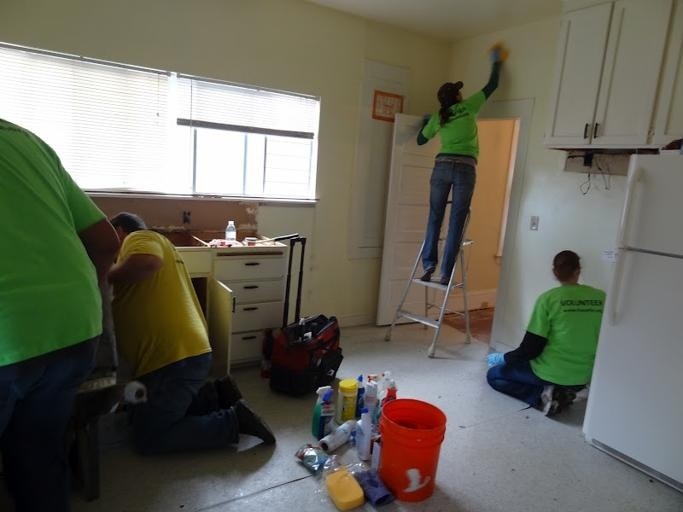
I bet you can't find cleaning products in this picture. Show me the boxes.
[356,373,365,415]
[382,371,399,408]
[356,406,373,463]
[365,374,381,416]
[319,419,358,453]
[311,384,337,441]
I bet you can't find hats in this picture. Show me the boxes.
[437,82,463,105]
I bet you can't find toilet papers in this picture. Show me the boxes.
[124,380,148,404]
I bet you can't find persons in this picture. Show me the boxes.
[413,42,504,288]
[0,116,121,512]
[104,210,277,457]
[481,248,606,419]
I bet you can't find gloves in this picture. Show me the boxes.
[487,353,506,368]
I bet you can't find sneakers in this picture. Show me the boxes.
[233,398,276,445]
[563,391,578,406]
[441,277,448,285]
[421,267,434,281]
[541,385,560,416]
[215,376,243,407]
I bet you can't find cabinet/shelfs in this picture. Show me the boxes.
[543,0,683,153]
[179,233,288,375]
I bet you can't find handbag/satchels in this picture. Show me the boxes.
[269,314,343,395]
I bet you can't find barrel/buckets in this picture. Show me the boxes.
[376,399,447,502]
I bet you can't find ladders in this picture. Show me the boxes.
[383,199,473,358]
